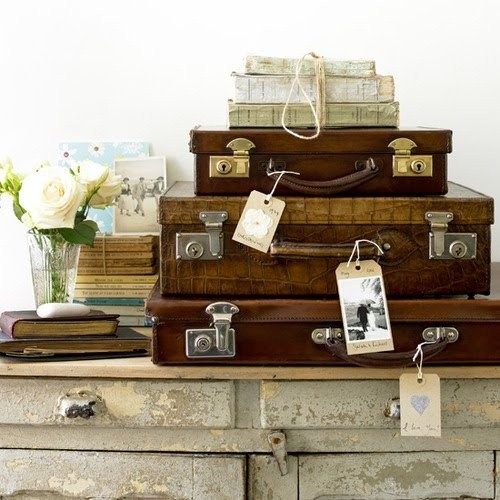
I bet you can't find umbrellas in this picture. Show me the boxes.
[357,299,376,306]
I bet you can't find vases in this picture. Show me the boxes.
[25,237,81,308]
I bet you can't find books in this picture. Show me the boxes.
[0,310,120,339]
[230,72,394,104]
[72,275,159,328]
[227,99,399,128]
[0,325,152,361]
[245,56,379,76]
[75,234,158,276]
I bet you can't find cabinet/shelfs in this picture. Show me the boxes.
[0,354,500,500]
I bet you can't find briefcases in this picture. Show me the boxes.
[146,276,499,368]
[157,181,494,297]
[187,125,453,195]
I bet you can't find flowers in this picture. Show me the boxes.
[0,154,116,303]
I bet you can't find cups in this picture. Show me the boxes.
[26,226,81,313]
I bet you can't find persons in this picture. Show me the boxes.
[151,177,165,220]
[366,305,376,330]
[357,304,370,332]
[132,177,148,216]
[118,177,135,216]
